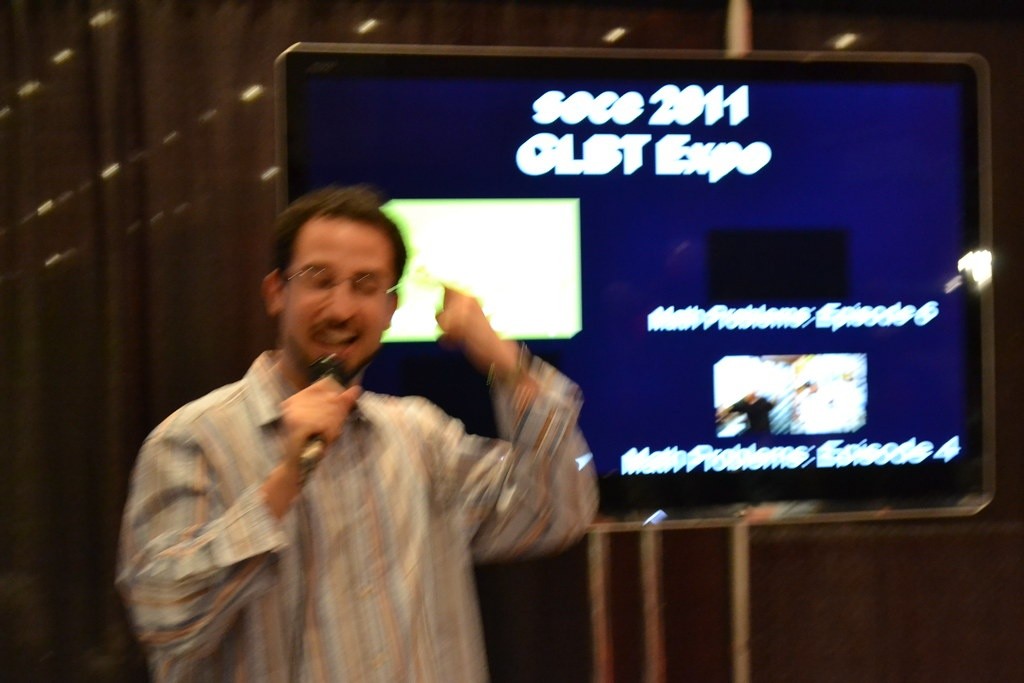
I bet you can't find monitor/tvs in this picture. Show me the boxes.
[280,54,994,533]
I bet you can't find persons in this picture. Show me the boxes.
[118,184,598,683]
[720,393,777,436]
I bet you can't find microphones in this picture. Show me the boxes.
[298,354,348,472]
